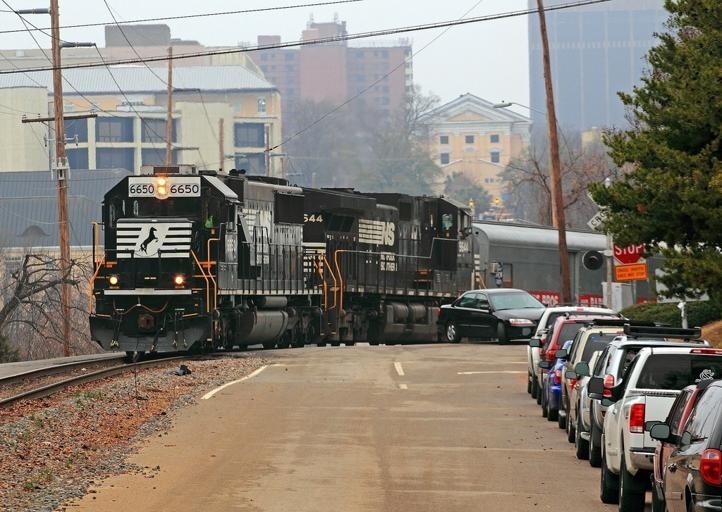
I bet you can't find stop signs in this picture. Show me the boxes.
[613,242,645,264]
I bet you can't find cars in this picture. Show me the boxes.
[437,288,546,344]
[527,307,722,512]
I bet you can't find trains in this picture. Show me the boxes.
[88,166,721,363]
